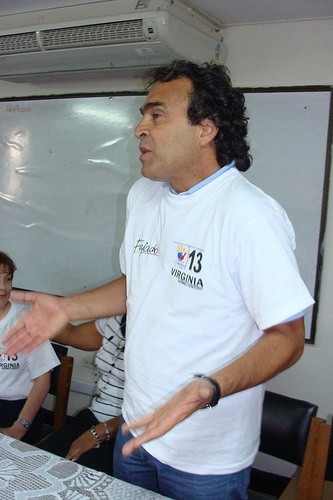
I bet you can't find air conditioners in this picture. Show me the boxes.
[0,0,227,81]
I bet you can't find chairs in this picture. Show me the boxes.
[23,342,75,448]
[248,389,333,500]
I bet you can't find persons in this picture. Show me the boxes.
[2,59,316,500]
[0,253,62,443]
[49,313,126,476]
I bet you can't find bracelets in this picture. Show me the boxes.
[90,427,101,448]
[102,421,110,440]
[17,418,30,429]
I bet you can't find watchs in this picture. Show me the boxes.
[194,374,220,409]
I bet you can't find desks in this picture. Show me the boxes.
[0,432,173,499]
